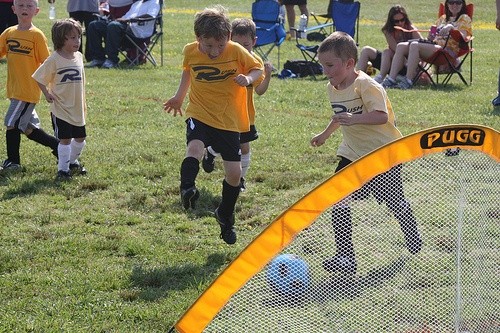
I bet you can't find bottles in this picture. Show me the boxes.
[49,2,55,19]
[299,13,307,38]
[431,25,436,38]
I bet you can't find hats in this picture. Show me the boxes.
[281,69,296,78]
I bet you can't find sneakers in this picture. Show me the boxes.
[239,177,246,192]
[323,260,357,275]
[57,170,72,181]
[202,148,216,173]
[180,187,199,211]
[51,152,59,163]
[214,209,237,245]
[0,161,21,170]
[405,233,422,254]
[382,78,397,88]
[394,80,413,88]
[69,164,86,174]
[374,77,383,83]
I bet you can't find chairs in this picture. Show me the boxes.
[92,0,163,67]
[252,0,287,75]
[291,0,360,80]
[409,3,473,89]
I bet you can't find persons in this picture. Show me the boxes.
[308,31,421,275]
[382,0,471,89]
[0,0,59,170]
[163,8,262,245]
[278,0,308,38]
[355,6,423,84]
[30,17,87,179]
[492,0,500,109]
[202,18,273,192]
[66,0,161,69]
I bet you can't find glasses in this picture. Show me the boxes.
[394,19,406,23]
[448,1,463,5]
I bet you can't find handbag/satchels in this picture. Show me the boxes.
[284,60,323,76]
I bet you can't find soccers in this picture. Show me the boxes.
[267,254,310,298]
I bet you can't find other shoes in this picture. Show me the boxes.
[493,96,500,105]
[101,60,119,69]
[84,60,104,68]
